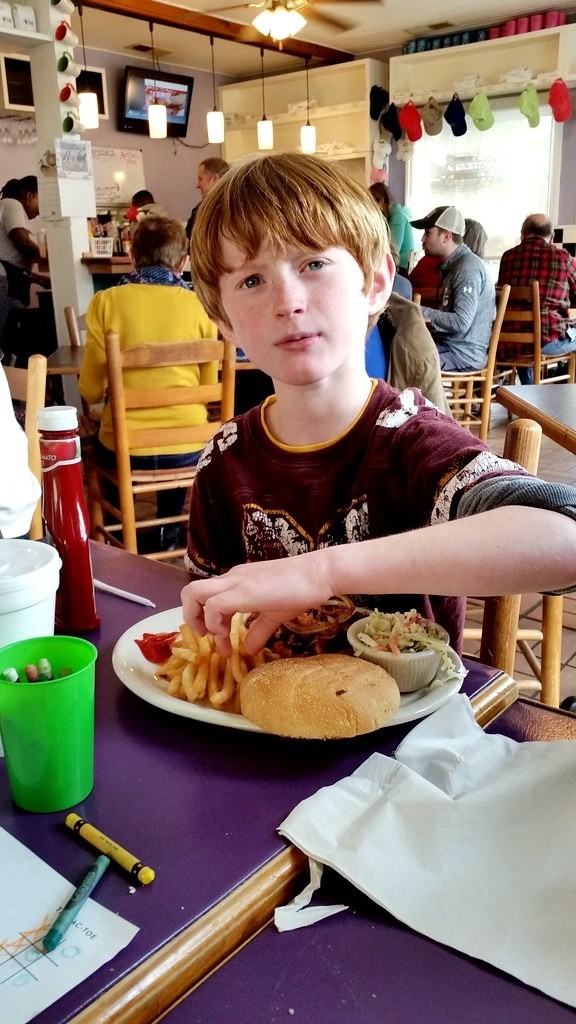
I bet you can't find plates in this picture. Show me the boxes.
[112,606,465,733]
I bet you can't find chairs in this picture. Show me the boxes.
[1,354,48,540]
[493,279,576,420]
[376,291,453,418]
[438,284,512,443]
[81,328,236,561]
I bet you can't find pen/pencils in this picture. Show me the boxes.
[92,578,155,608]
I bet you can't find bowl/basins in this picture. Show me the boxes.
[348,615,450,693]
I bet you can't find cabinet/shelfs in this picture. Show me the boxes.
[217,58,389,188]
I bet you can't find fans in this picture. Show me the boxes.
[178,0,384,43]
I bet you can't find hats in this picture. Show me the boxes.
[370,167,388,184]
[372,138,392,171]
[410,205,466,237]
[469,90,494,131]
[370,85,390,121]
[381,103,402,143]
[397,136,415,161]
[419,95,443,135]
[443,92,467,136]
[518,83,540,128]
[548,77,572,122]
[399,100,423,141]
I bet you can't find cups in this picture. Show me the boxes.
[0,539,63,647]
[1,635,99,813]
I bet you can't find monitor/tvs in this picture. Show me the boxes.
[119,66,195,138]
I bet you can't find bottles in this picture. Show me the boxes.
[121,222,131,257]
[37,405,101,629]
[38,227,48,258]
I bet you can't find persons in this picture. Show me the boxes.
[0,363,41,539]
[145,88,184,116]
[497,213,576,384]
[77,217,218,551]
[408,219,488,330]
[0,175,46,367]
[179,151,576,661]
[129,190,167,238]
[186,157,229,259]
[409,206,494,373]
[369,182,413,277]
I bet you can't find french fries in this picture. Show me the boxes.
[154,611,268,714]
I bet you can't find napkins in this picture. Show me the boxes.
[276,692,576,1008]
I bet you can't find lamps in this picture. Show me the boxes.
[147,21,167,140]
[77,4,100,130]
[252,0,311,42]
[256,48,273,150]
[205,36,224,143]
[299,59,316,153]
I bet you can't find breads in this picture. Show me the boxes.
[238,653,399,739]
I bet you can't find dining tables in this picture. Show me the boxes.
[148,694,576,1024]
[0,537,518,1024]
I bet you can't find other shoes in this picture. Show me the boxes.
[153,521,185,561]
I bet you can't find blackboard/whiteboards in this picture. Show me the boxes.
[92,145,147,207]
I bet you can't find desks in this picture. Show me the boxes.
[496,384,576,456]
[46,344,259,376]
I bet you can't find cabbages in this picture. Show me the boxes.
[358,610,469,692]
